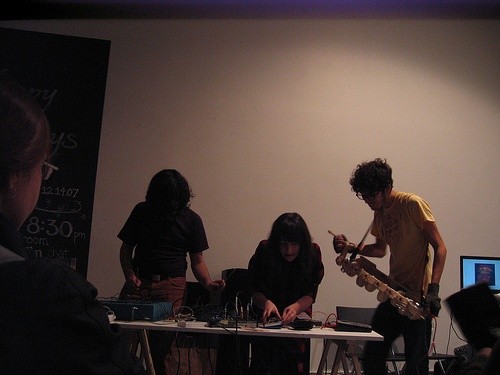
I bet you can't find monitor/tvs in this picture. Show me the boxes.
[460,256,500,294]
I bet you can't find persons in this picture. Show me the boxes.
[0,77,141,375]
[247,212,325,375]
[333,159,447,375]
[117,168,226,375]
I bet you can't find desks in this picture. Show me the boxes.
[109,315,384,375]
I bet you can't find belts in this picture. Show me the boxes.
[134,269,185,281]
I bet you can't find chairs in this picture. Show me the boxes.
[177,282,228,349]
[221,267,261,319]
[336,306,409,374]
[422,341,462,375]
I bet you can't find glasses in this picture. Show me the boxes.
[356,190,381,201]
[36,157,59,179]
